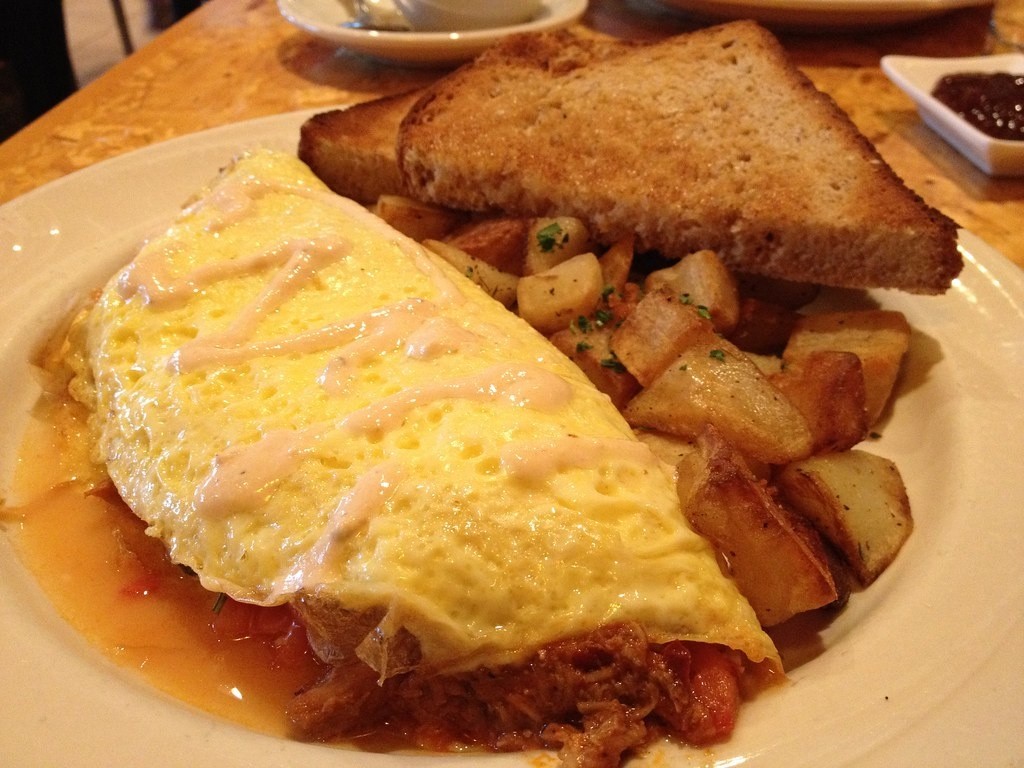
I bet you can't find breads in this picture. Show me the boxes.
[297,86,425,203]
[398,20,963,296]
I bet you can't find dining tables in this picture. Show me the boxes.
[0,0,1024,269]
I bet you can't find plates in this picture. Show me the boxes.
[278,0,590,62]
[2,101,1024,768]
[880,53,1024,175]
